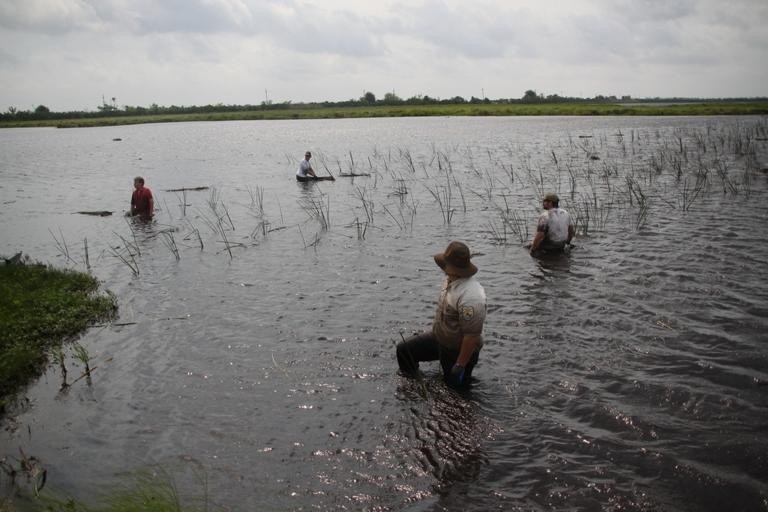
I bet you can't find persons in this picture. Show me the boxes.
[295,151,317,182]
[522,191,576,254]
[396,241,486,385]
[402,395,486,508]
[130,176,153,218]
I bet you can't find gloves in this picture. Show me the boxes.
[313,175,318,180]
[529,245,539,258]
[446,363,466,387]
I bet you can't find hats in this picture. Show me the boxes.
[537,192,559,202]
[304,151,311,157]
[434,241,478,278]
